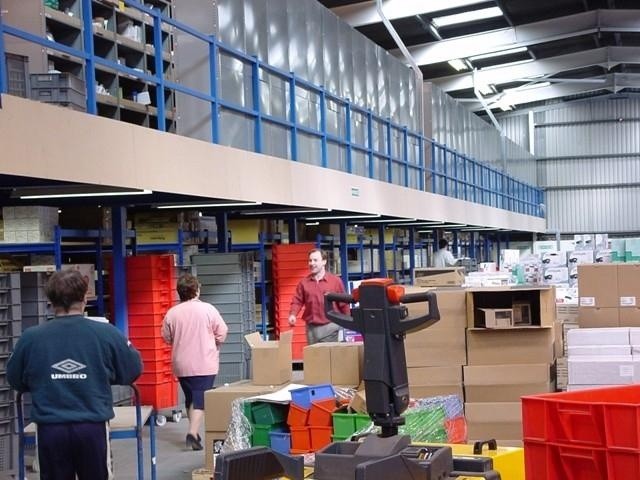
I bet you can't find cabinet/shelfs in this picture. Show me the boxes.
[41,0,176,134]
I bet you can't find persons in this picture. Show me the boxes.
[6,269,144,480]
[432,238,460,267]
[287,248,351,345]
[161,273,228,451]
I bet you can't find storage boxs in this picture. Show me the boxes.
[30,73,87,113]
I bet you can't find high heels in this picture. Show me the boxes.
[186,433,202,451]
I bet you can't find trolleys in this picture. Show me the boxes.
[13,382,163,480]
[132,400,182,426]
